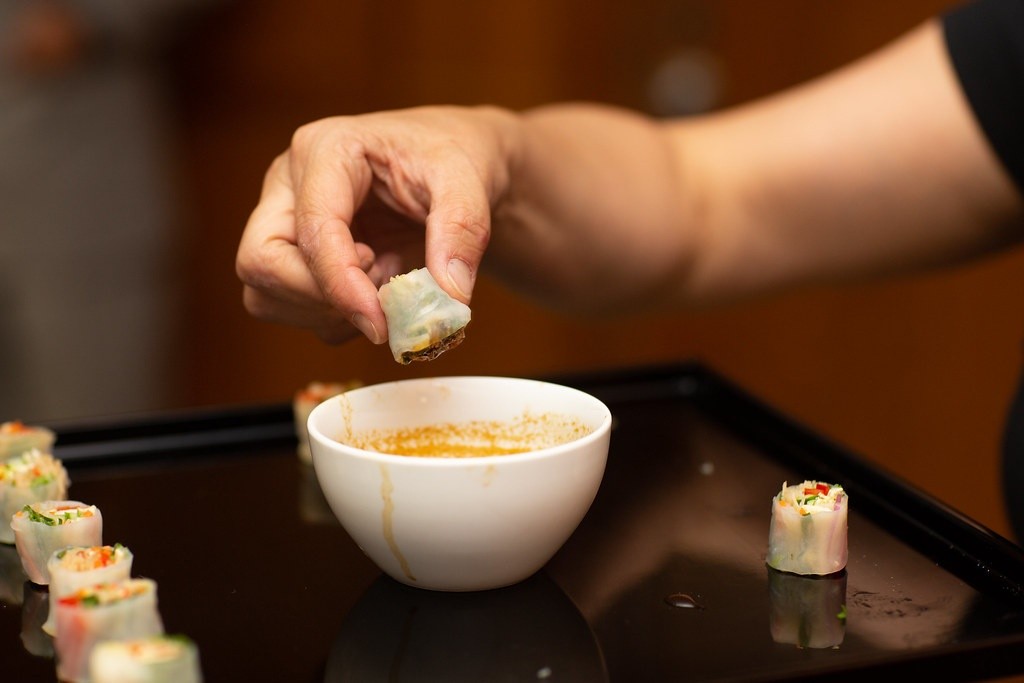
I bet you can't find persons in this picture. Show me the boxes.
[235,0,1024,553]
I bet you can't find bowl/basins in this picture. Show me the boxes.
[306,377,611,591]
